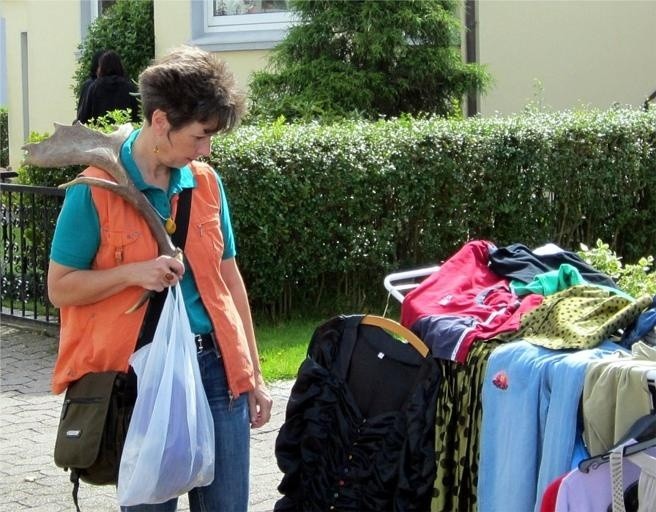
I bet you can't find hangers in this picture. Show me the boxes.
[361,289,428,358]
[577,414,656,475]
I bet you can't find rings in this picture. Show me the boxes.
[163,270,175,284]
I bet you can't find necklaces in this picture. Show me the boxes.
[138,189,177,237]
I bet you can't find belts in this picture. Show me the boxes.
[193,334,214,355]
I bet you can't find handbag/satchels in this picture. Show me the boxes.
[54,364,137,486]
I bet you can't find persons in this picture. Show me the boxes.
[45,45,274,512]
[71,49,140,125]
[75,49,103,117]
